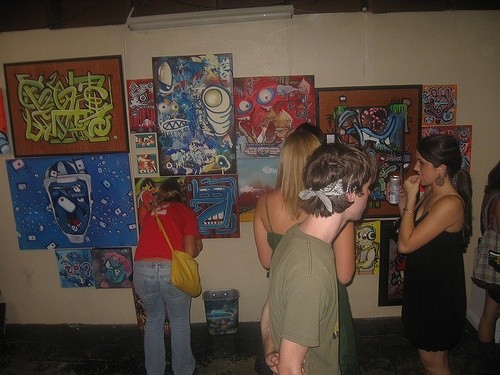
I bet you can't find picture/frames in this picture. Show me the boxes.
[315,84,422,218]
[3,54,130,158]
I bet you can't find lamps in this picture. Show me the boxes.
[127,4,294,31]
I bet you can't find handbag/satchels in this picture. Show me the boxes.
[172,250,202,298]
[471,195,500,291]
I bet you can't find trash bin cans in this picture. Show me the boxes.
[203,289,241,335]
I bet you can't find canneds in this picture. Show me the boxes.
[389,175,400,204]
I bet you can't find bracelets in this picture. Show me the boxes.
[404,208,414,216]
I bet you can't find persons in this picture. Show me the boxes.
[385,134,472,375]
[133,177,203,375]
[253,129,359,375]
[472,161,500,375]
[259,140,379,375]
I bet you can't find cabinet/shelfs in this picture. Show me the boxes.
[378,220,408,307]
